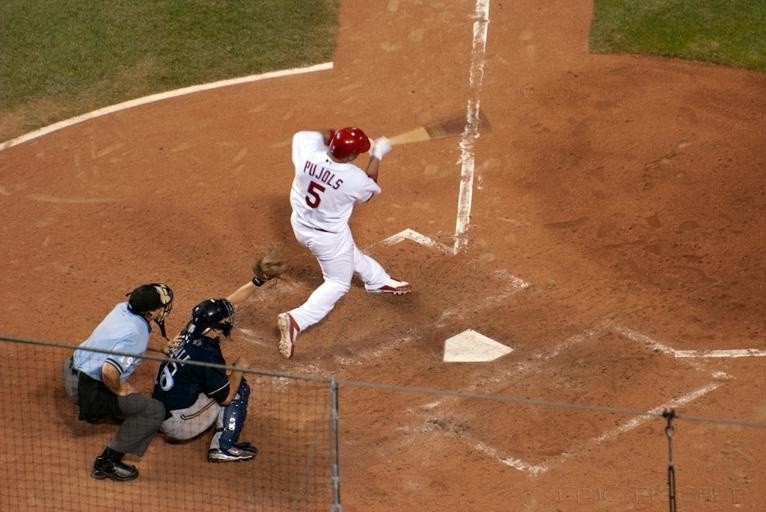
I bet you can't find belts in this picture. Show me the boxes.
[69,355,77,376]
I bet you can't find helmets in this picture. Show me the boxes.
[192,297,235,335]
[329,127,371,160]
[124,282,174,312]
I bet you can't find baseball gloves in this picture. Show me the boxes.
[253,254,288,282]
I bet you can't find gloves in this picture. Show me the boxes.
[367,136,392,161]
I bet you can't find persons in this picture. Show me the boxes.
[157,254,289,461]
[276,127,411,359]
[63,284,184,481]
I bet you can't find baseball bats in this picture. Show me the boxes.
[388,108,491,154]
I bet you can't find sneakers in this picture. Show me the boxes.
[208,447,253,463]
[277,312,299,359]
[368,277,412,296]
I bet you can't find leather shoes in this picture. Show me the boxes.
[92,456,139,481]
